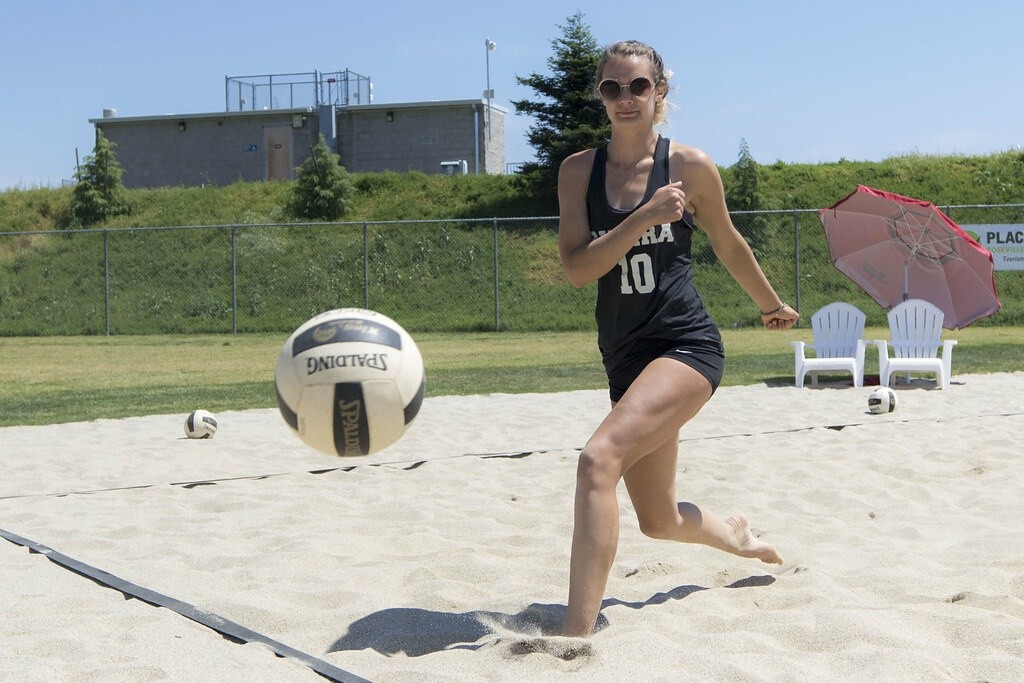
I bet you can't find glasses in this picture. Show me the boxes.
[596,76,657,101]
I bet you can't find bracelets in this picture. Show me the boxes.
[761,302,786,316]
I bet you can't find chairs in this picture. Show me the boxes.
[873,299,958,390]
[790,301,873,388]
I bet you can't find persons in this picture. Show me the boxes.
[558,39,800,638]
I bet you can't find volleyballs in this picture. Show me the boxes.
[274,306,426,458]
[868,387,900,414]
[184,409,217,439]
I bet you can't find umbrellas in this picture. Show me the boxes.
[817,183,1003,383]
